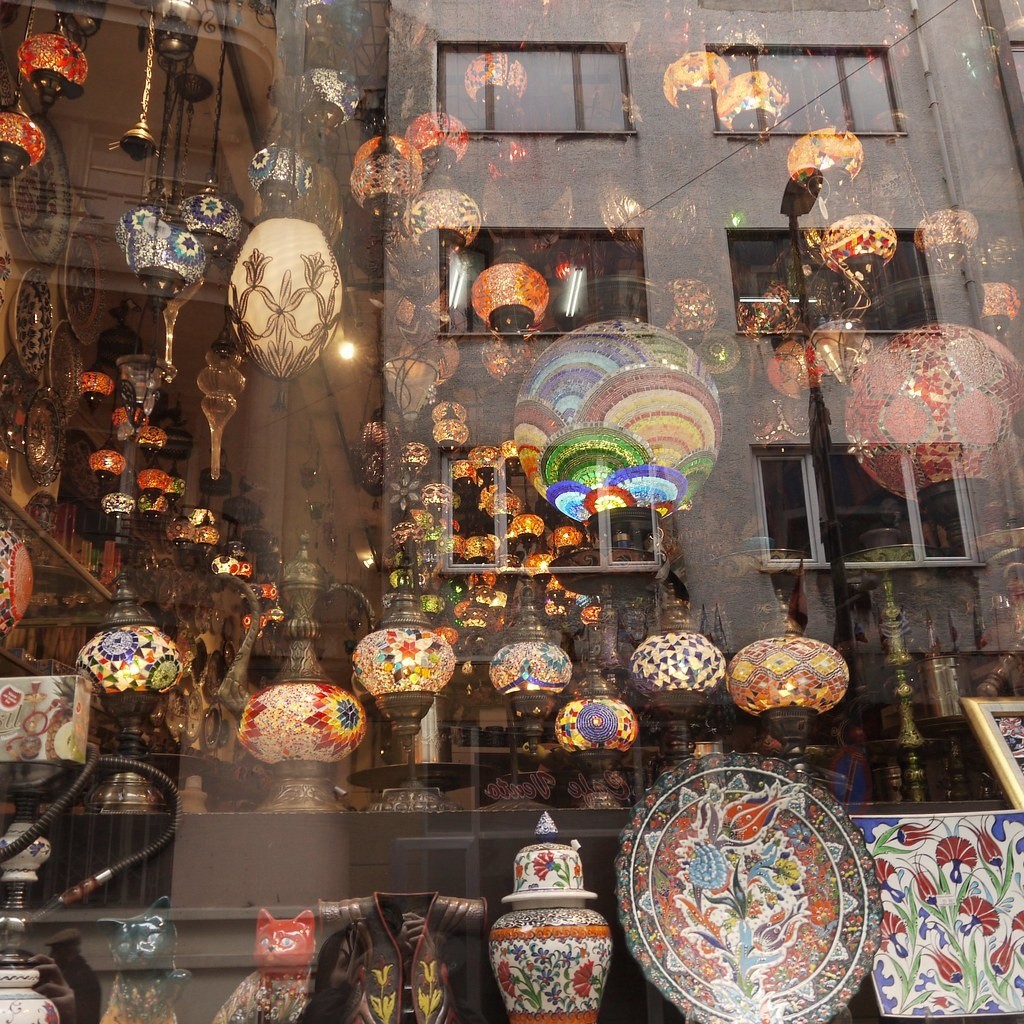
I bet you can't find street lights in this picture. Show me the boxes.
[778,165,866,721]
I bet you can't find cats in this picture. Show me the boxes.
[212,909,316,1024]
[97,896,190,1024]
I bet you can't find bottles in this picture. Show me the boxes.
[488,811,613,1024]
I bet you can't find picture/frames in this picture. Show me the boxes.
[959,694,1024,811]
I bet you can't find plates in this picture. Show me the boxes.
[614,750,880,1024]
[849,809,1024,1017]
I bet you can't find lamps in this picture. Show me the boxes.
[0,0,1024,811]
[740,296,818,304]
[449,261,467,310]
[565,268,584,318]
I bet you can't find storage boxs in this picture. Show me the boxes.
[0,674,93,766]
[8,647,40,670]
[38,658,77,676]
